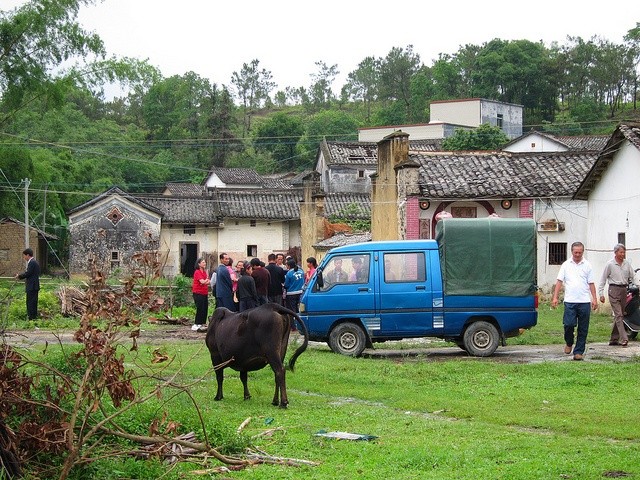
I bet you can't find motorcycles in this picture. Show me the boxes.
[610,267,640,341]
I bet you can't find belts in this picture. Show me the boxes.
[610,284,628,287]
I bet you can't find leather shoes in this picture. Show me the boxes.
[574,354,583,360]
[565,346,572,353]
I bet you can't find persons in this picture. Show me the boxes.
[259,261,265,267]
[216,253,234,312]
[276,253,287,270]
[600,243,640,347]
[15,249,40,319]
[264,254,285,304]
[384,260,395,281]
[192,258,210,330]
[250,258,268,304]
[235,260,245,277]
[283,257,304,330]
[324,256,348,286]
[210,268,217,297]
[236,262,258,312]
[281,258,294,307]
[226,257,239,302]
[350,257,362,281]
[304,257,318,285]
[551,242,598,360]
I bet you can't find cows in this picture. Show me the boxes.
[205,303,309,409]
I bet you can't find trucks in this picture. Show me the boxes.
[294,218,539,358]
